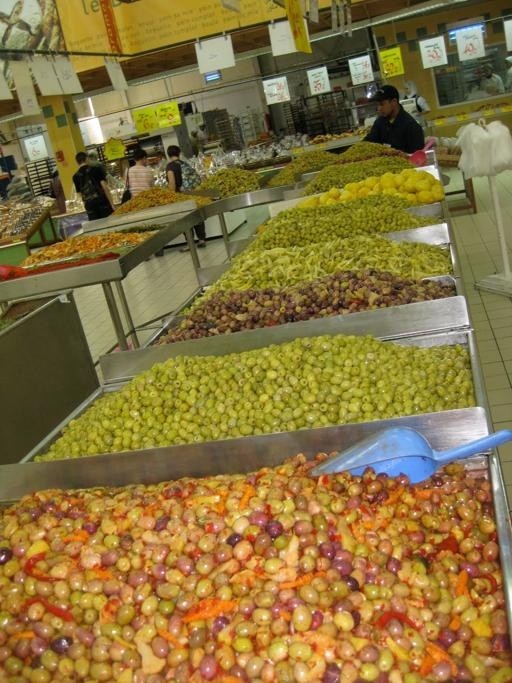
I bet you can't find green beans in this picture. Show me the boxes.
[179,192,453,316]
[34,335,476,462]
[0,452,512,683]
[110,186,213,206]
[278,141,416,195]
[195,168,262,198]
[149,267,456,346]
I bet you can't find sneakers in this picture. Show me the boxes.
[179,242,207,252]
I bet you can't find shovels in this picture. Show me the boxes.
[304,427,512,486]
[410,139,436,166]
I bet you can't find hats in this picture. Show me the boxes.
[367,85,398,102]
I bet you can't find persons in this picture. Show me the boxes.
[191,123,209,157]
[73,150,116,221]
[480,56,512,96]
[361,79,430,154]
[51,170,66,214]
[166,146,206,252]
[121,150,163,261]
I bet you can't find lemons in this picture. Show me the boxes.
[296,168,446,208]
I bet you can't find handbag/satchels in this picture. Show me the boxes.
[119,189,132,206]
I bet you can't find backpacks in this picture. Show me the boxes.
[174,160,201,196]
[76,167,101,202]
